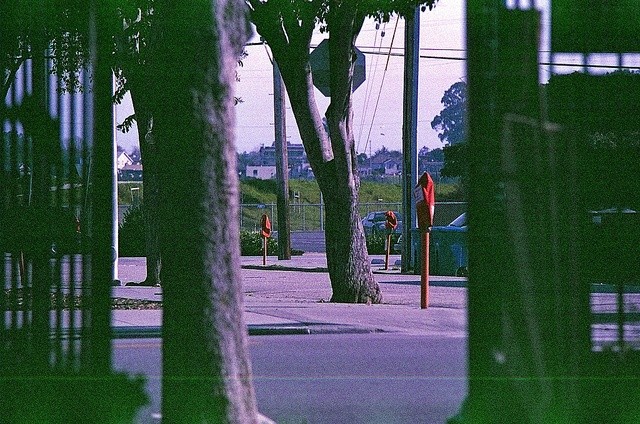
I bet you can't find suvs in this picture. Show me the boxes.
[0,206,81,258]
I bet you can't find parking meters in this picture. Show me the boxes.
[385,210,398,270]
[413,173,434,308]
[260,214,272,263]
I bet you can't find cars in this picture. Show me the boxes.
[395,211,468,264]
[362,211,403,240]
